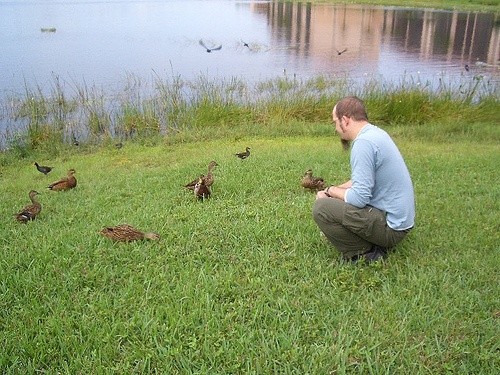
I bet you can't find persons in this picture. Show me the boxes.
[313,94,416,260]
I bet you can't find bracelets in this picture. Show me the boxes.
[325,185,335,199]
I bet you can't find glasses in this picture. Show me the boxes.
[330,116,350,126]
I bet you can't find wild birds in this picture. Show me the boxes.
[199,41,222,53]
[12,190,42,224]
[300,170,324,191]
[194,174,212,200]
[33,161,55,175]
[241,41,250,48]
[97,224,161,242]
[46,169,80,192]
[182,161,219,190]
[235,147,251,159]
[333,47,347,56]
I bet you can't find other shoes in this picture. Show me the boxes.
[352,246,386,264]
